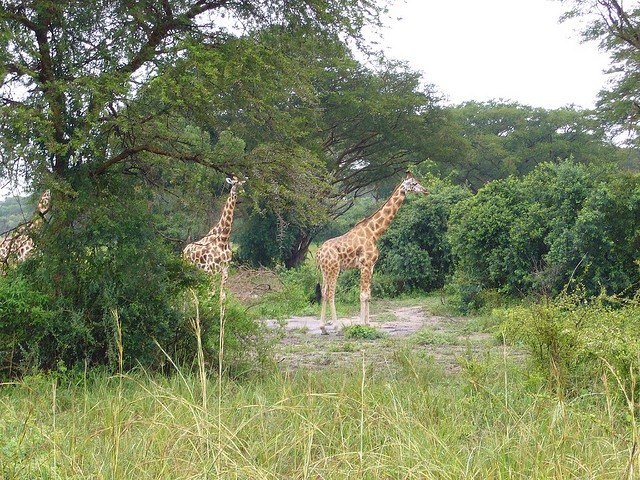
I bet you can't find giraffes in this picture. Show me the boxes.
[315,169,430,336]
[0,183,61,278]
[181,172,248,302]
